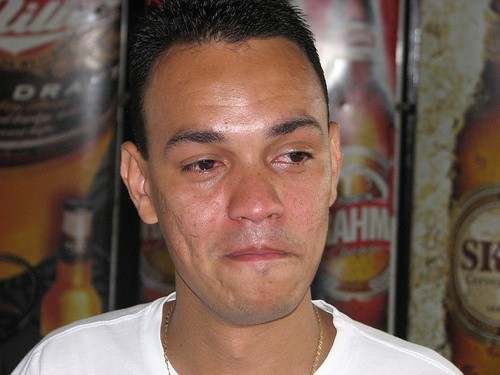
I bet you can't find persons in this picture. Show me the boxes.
[9,1,466,375]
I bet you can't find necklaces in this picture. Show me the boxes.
[163,301,323,375]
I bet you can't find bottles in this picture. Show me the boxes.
[440,0,500,375]
[38,198,101,344]
[304,1,397,341]
